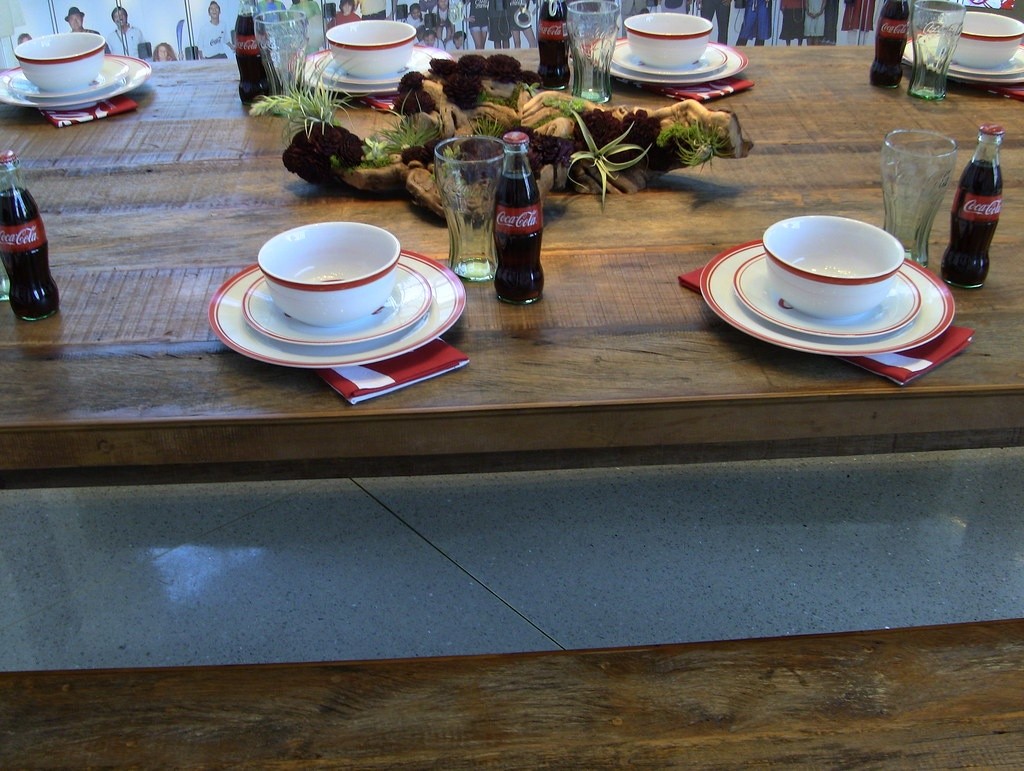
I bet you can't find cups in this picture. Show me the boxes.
[434,134,505,282]
[566,0,621,105]
[0,159,26,300]
[880,129,958,268]
[907,0,966,101]
[253,10,306,98]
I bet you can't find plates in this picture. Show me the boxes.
[0,54,152,111]
[898,40,1024,85]
[207,250,466,369]
[588,37,748,86]
[699,241,956,357]
[301,44,454,95]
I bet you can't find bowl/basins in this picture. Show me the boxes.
[256,222,402,329]
[624,12,714,68]
[325,20,417,78]
[13,32,105,92]
[761,215,905,322]
[938,12,1024,70]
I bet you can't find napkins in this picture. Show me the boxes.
[902,39,1023,103]
[613,78,769,105]
[675,266,982,388]
[316,334,472,405]
[38,95,138,130]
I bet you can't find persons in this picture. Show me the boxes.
[198,1,235,59]
[152,42,177,62]
[18,33,32,45]
[621,0,732,44]
[405,0,466,50]
[462,0,539,49]
[253,0,398,52]
[841,0,876,46]
[65,6,111,54]
[736,0,826,46]
[107,7,143,58]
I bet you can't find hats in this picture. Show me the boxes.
[65,7,85,21]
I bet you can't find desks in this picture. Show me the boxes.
[1,38,1024,495]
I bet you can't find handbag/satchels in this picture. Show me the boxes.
[734,0,746,8]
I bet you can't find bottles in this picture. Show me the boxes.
[870,0,910,89]
[493,131,545,304]
[234,0,281,107]
[940,122,1006,289]
[536,0,571,91]
[0,151,59,320]
